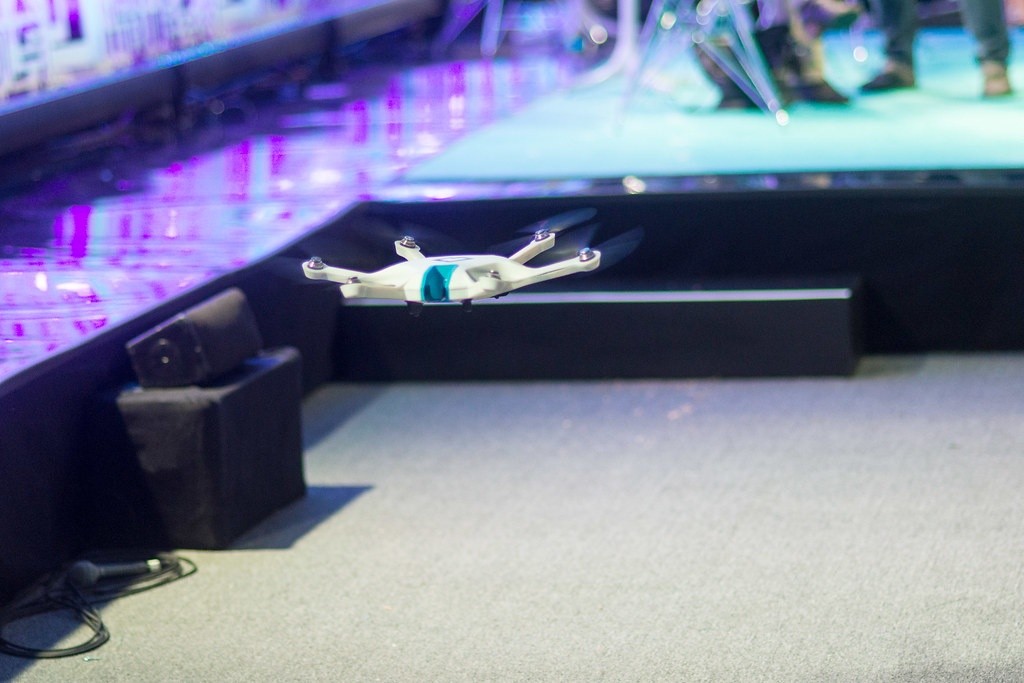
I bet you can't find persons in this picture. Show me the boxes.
[694,0,847,112]
[856,0,1011,100]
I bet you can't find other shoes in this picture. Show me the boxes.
[795,81,848,103]
[719,93,755,108]
[983,60,1013,97]
[860,61,914,92]
